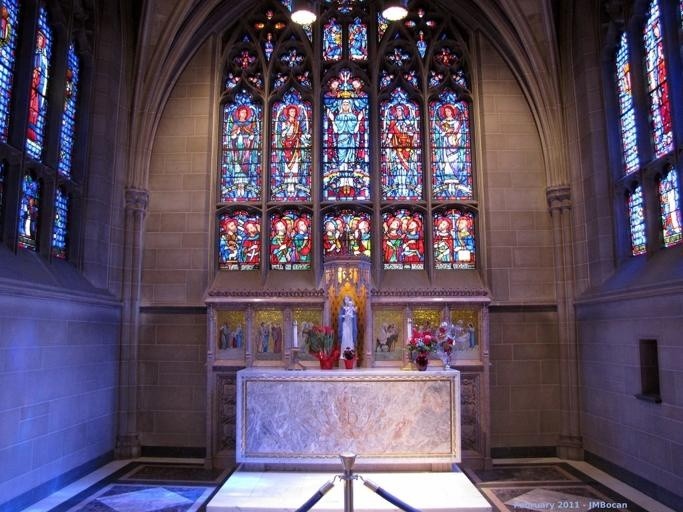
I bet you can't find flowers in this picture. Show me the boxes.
[407,322,458,357]
[303,323,355,359]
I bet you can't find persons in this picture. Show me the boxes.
[278,104,308,194]
[339,296,359,359]
[434,107,467,194]
[326,97,366,174]
[217,316,477,352]
[227,106,255,177]
[221,211,475,266]
[383,103,419,199]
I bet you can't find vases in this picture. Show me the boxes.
[318,356,354,369]
[412,351,428,371]
